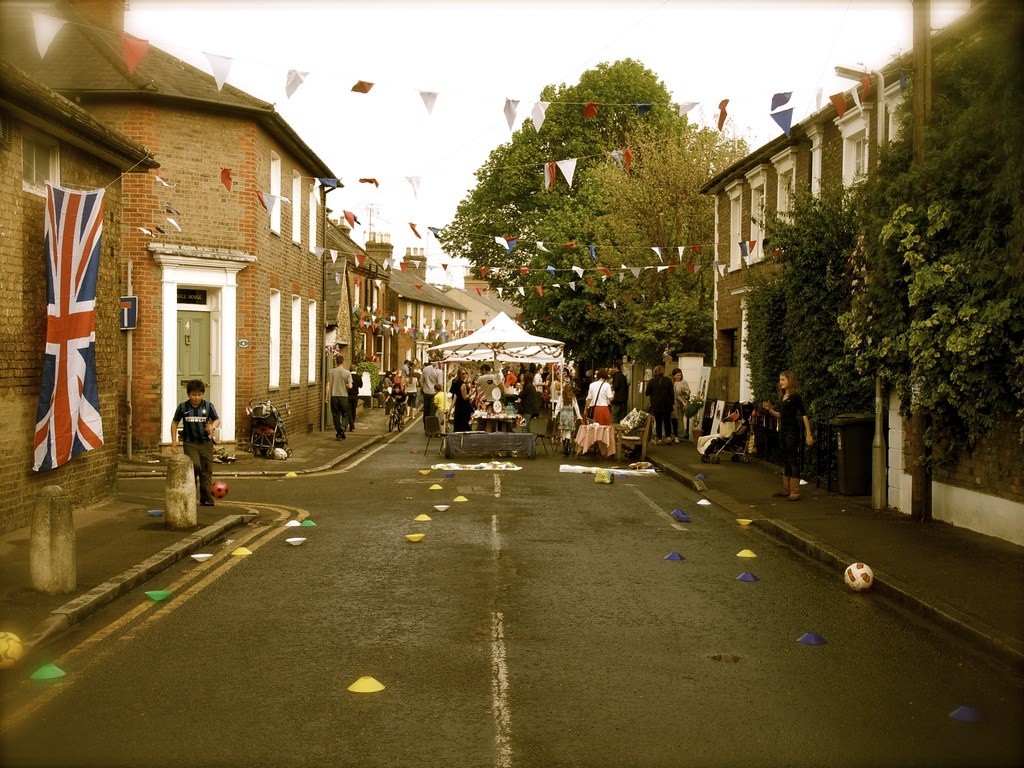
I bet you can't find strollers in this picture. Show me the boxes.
[696,400,757,464]
[247,396,292,459]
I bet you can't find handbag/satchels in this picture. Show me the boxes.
[586,406,595,419]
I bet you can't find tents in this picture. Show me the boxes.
[435,346,564,420]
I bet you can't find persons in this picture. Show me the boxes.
[325,355,363,441]
[504,373,520,433]
[171,380,221,508]
[473,364,504,433]
[645,365,675,446]
[517,363,629,428]
[449,367,476,433]
[762,370,813,501]
[419,363,444,437]
[611,362,628,423]
[670,368,691,444]
[552,383,583,456]
[373,357,443,428]
[434,384,451,437]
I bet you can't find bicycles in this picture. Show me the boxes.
[385,401,405,434]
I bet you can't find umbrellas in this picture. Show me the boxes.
[426,310,565,373]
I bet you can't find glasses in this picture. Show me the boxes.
[462,372,468,375]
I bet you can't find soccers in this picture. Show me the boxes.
[844,562,874,592]
[211,481,228,498]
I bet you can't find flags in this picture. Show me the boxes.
[31,178,107,473]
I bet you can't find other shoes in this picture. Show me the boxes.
[665,438,672,444]
[653,440,662,445]
[350,428,355,432]
[674,436,680,444]
[335,434,341,438]
[201,500,213,506]
[339,428,346,440]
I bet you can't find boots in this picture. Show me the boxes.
[773,474,791,497]
[787,477,801,501]
[561,438,570,455]
